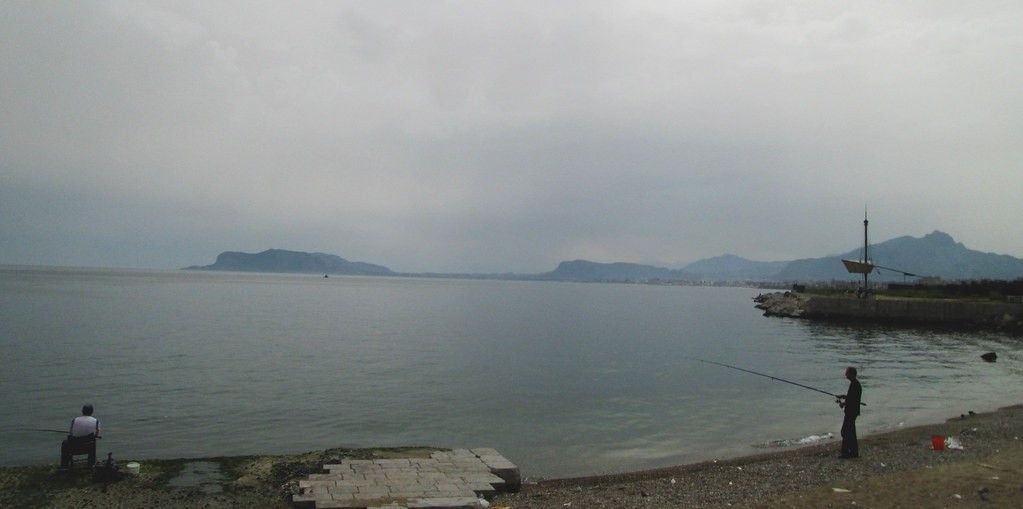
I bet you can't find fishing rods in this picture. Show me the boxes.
[686,354,866,407]
[1,429,102,438]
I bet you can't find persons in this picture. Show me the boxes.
[836,366,862,460]
[857,287,867,298]
[59,403,102,470]
[865,288,875,299]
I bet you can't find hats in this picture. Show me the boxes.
[83,403,93,411]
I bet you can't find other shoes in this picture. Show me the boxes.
[89,461,97,468]
[849,454,859,458]
[57,465,69,470]
[842,455,849,458]
